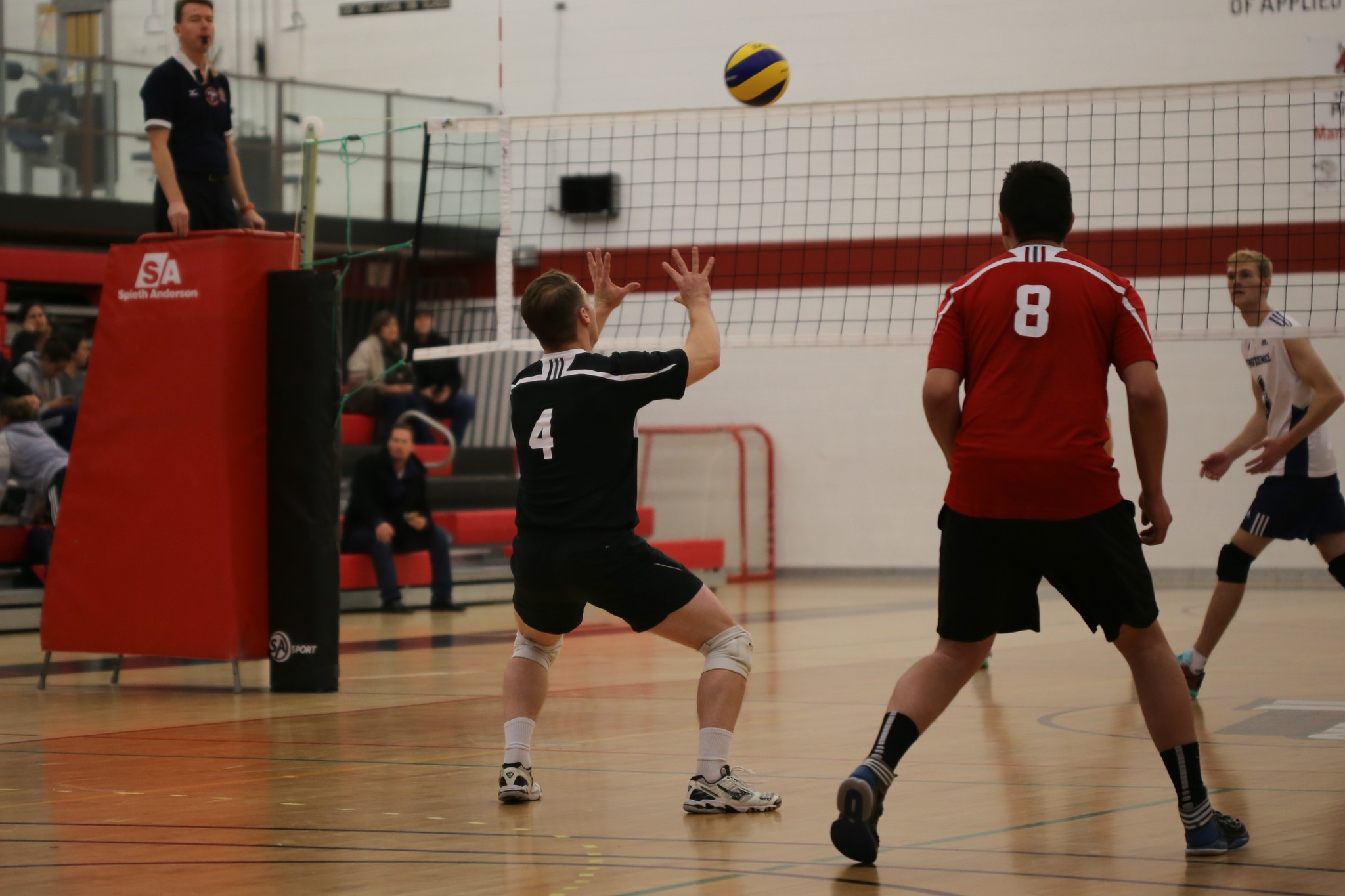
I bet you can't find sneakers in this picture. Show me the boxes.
[498,761,542,803]
[1184,807,1249,856]
[683,765,781,814]
[1173,649,1206,697]
[831,764,886,863]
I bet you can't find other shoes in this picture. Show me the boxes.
[430,597,465,612]
[380,600,414,614]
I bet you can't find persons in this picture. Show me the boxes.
[1173,250,1345,698]
[1,0,477,614]
[830,159,1251,865]
[499,246,784,816]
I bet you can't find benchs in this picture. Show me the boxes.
[0,345,724,591]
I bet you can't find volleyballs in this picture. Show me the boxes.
[724,41,790,106]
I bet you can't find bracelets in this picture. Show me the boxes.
[240,202,254,213]
